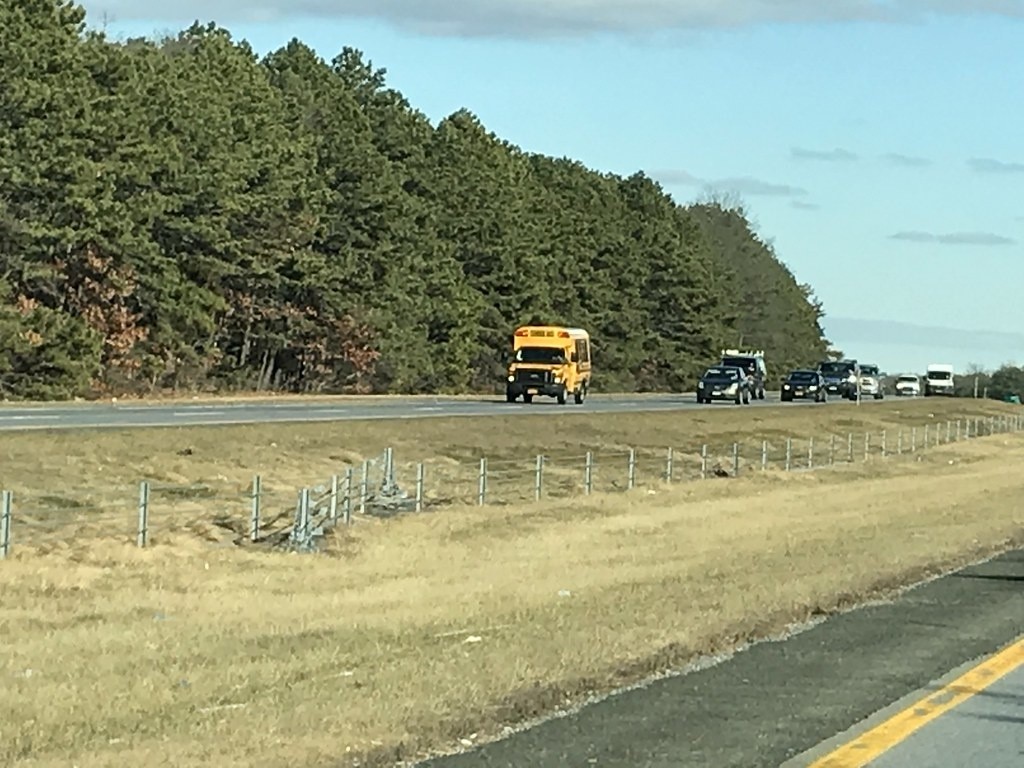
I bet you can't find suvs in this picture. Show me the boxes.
[719,348,767,400]
[816,360,862,402]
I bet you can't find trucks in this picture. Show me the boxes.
[923,365,956,396]
[504,325,591,405]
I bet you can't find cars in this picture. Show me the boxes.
[780,370,829,403]
[695,366,754,405]
[895,375,920,396]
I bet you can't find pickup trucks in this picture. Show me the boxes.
[858,363,886,400]
